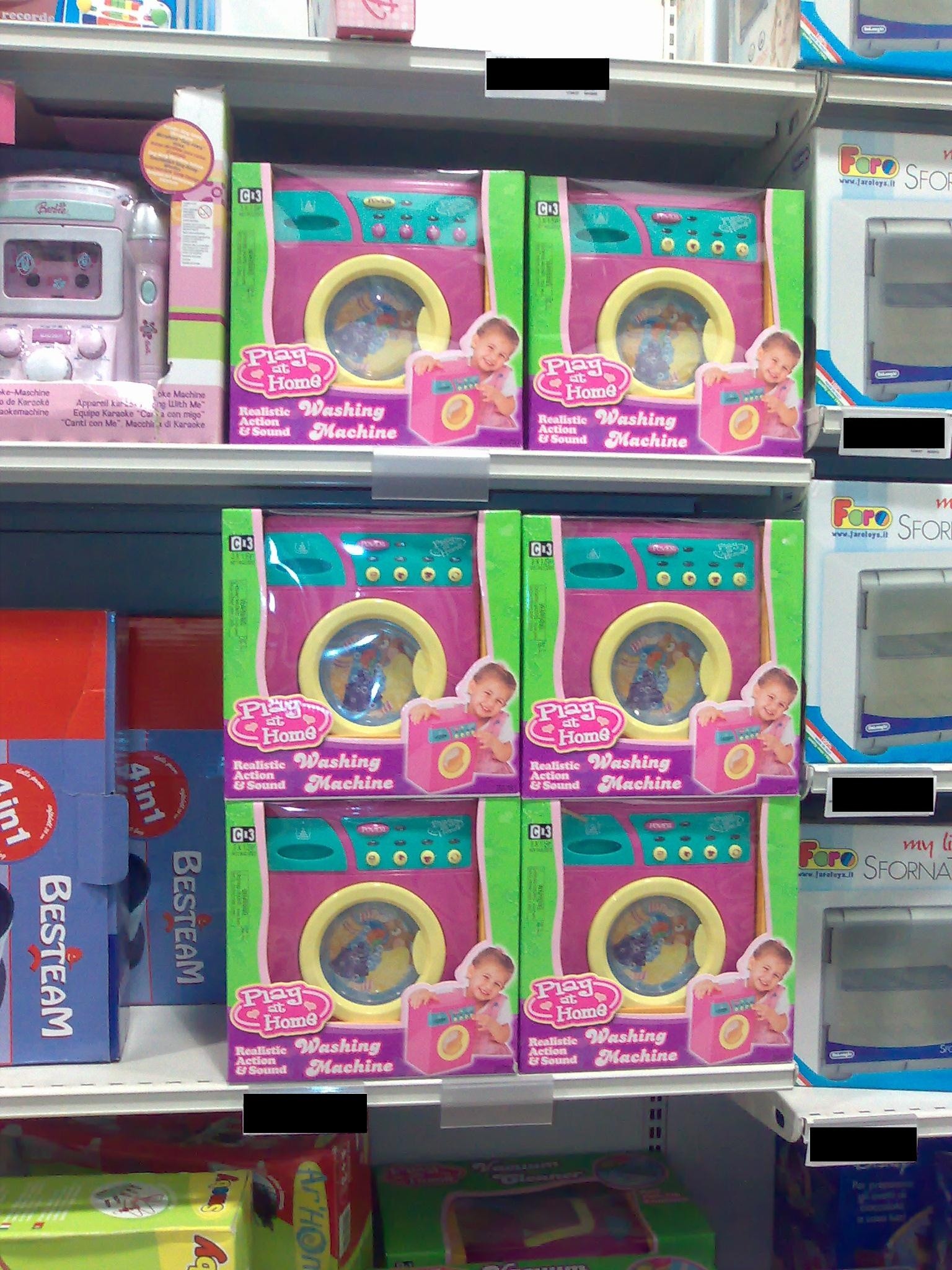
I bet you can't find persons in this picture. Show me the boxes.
[695,667,799,778]
[692,940,792,1045]
[412,317,520,430]
[408,946,514,1056]
[700,332,802,442]
[407,661,517,778]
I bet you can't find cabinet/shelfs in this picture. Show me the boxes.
[0,0,952,1270]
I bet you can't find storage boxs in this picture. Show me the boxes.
[120,616,229,1005]
[519,515,805,802]
[520,799,800,1077]
[312,0,417,42]
[0,608,132,1065]
[0,0,217,31]
[220,508,524,803]
[228,159,529,452]
[792,821,952,1096]
[0,1111,374,1270]
[773,479,952,765]
[727,0,952,82]
[762,125,952,412]
[222,796,522,1084]
[771,1109,952,1270]
[521,173,808,461]
[0,1169,255,1270]
[371,1146,719,1270]
[0,79,237,444]
